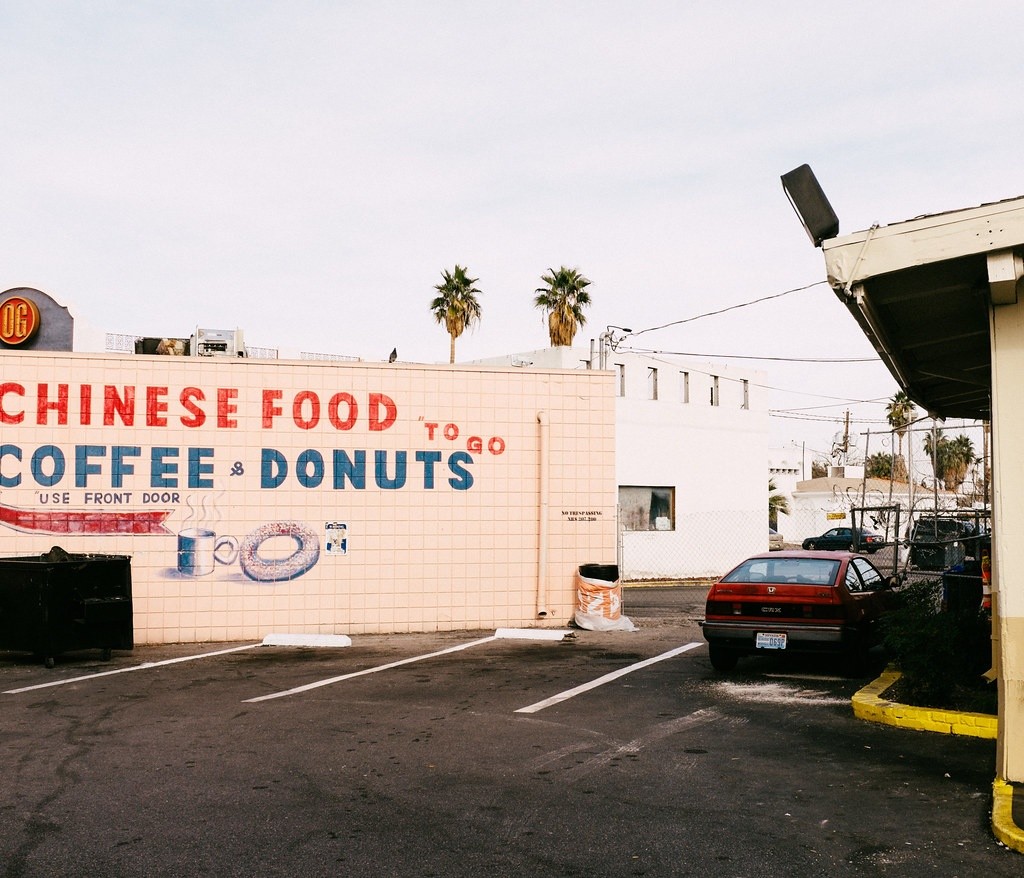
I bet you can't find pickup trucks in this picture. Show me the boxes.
[905,519,992,566]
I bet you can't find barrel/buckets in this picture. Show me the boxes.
[579,564,619,630]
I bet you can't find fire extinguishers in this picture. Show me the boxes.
[978,556,992,609]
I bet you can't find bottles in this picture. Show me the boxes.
[944,532,965,572]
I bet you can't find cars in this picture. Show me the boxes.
[699,550,902,672]
[802,527,885,555]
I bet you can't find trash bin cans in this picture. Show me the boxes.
[0,553,135,669]
[940,565,983,616]
[577,564,620,630]
[908,519,967,568]
